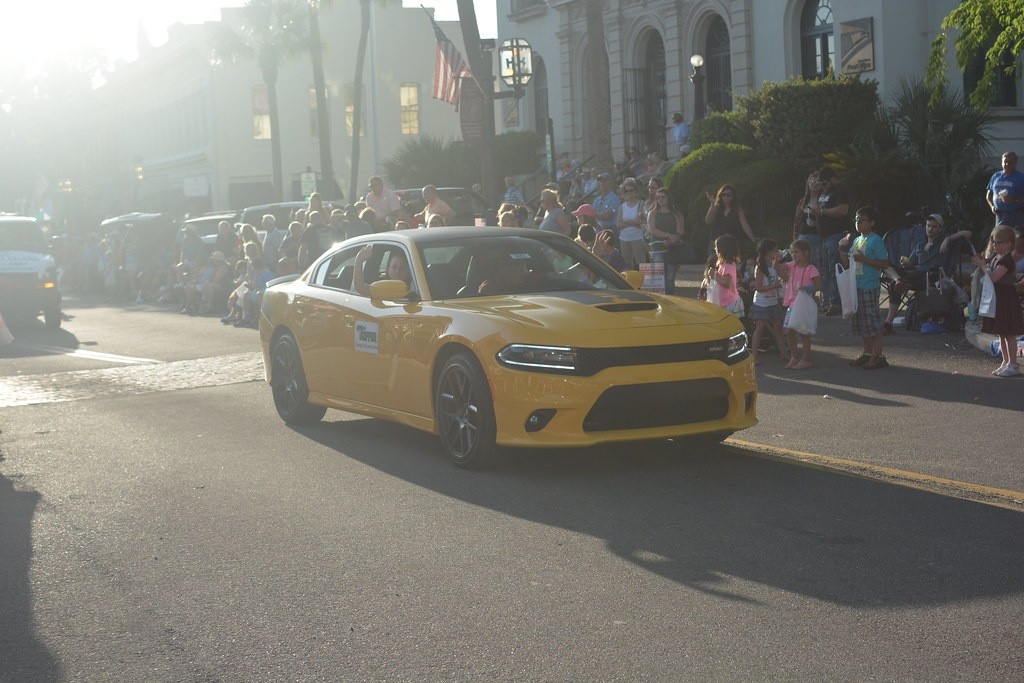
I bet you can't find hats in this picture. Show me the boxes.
[597,172,612,180]
[571,204,596,217]
[544,182,560,190]
[927,214,945,224]
[367,176,383,187]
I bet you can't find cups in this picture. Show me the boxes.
[998,190,1008,204]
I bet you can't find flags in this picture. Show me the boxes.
[429,18,473,114]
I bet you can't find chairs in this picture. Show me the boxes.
[880,276,918,318]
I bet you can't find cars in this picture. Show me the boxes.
[257,226,760,471]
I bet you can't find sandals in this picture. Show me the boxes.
[792,359,813,369]
[786,357,799,369]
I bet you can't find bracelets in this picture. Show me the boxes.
[819,209,823,216]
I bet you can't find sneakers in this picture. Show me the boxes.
[992,361,1007,376]
[855,353,870,366]
[863,353,889,369]
[998,362,1020,377]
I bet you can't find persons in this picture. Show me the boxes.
[477,231,612,295]
[749,236,793,366]
[571,172,686,296]
[971,152,1024,377]
[47,223,146,298]
[883,214,971,335]
[701,184,760,256]
[772,241,822,372]
[837,208,890,368]
[790,168,853,316]
[697,233,750,322]
[351,245,420,298]
[466,145,642,232]
[705,103,717,116]
[670,112,691,158]
[279,174,457,328]
[152,210,283,326]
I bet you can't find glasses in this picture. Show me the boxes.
[721,192,733,197]
[821,183,828,186]
[809,180,820,185]
[625,188,635,192]
[852,219,870,222]
[991,239,1010,246]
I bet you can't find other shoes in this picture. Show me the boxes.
[894,281,911,293]
[883,323,894,335]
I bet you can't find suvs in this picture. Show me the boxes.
[0,213,65,329]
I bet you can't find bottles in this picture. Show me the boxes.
[744,269,749,280]
[901,256,909,266]
[784,307,791,327]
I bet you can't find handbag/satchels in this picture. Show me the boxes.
[788,281,818,334]
[835,254,858,320]
[979,273,996,318]
[706,271,720,305]
